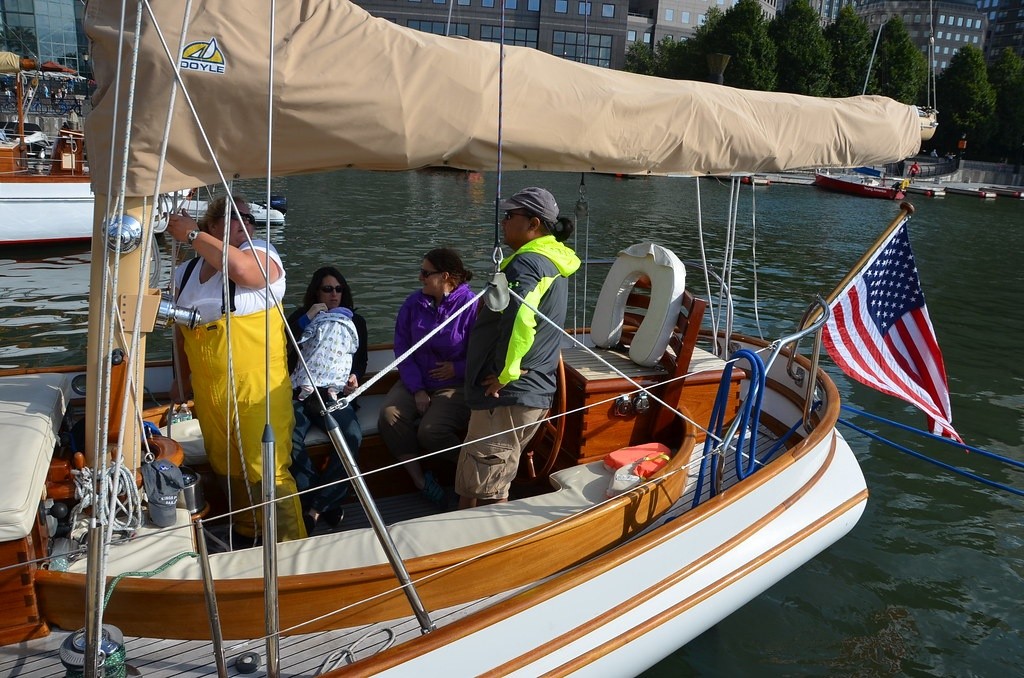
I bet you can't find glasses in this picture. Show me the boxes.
[420,268,450,278]
[505,211,542,223]
[215,210,256,226]
[316,285,342,293]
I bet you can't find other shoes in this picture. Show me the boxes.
[302,505,320,534]
[320,501,346,527]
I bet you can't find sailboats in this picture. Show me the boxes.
[0,0,937,678]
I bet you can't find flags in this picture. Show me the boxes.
[821,219,969,454]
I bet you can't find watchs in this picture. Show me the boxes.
[188,230,201,245]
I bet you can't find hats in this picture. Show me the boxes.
[492,187,559,225]
[141,459,185,526]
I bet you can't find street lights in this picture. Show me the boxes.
[82,49,90,100]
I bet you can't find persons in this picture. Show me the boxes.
[163,198,308,545]
[289,266,368,535]
[4,77,74,110]
[379,249,479,511]
[454,187,582,508]
[299,307,353,401]
[909,161,919,184]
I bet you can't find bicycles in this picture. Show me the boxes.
[0,97,19,113]
[54,98,83,116]
[24,98,48,114]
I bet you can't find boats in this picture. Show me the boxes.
[0,51,200,243]
[604,173,635,178]
[815,172,907,200]
[177,201,285,225]
[761,174,816,185]
[714,175,770,184]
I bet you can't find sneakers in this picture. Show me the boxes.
[422,472,451,513]
